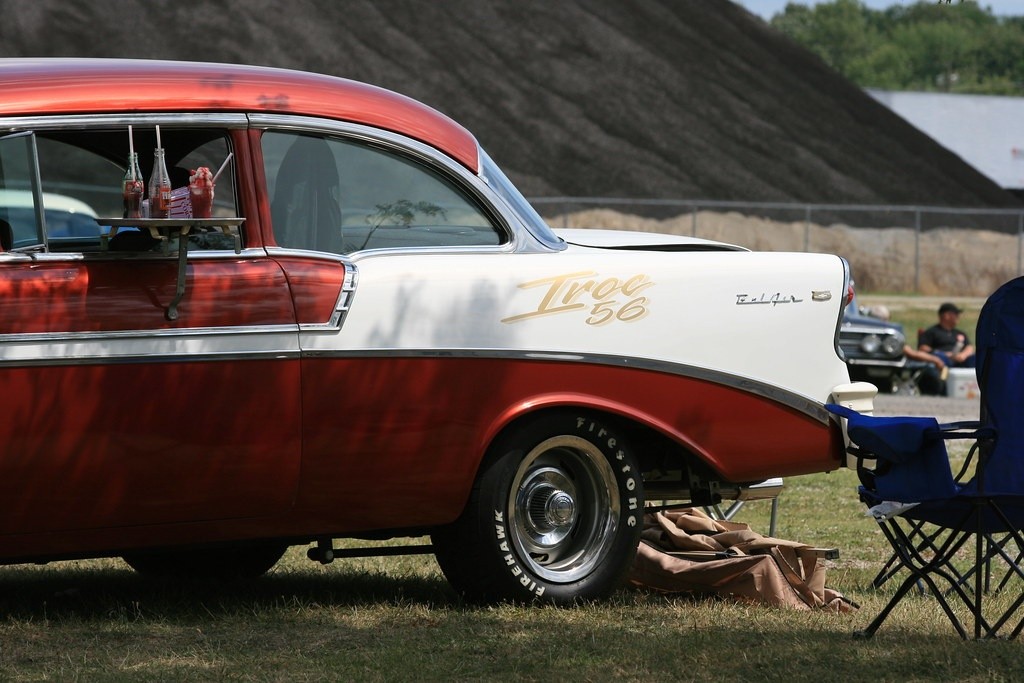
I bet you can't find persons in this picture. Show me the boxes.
[869,304,946,397]
[917,301,976,369]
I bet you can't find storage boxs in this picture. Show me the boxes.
[947,367,981,398]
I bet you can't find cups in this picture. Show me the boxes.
[188,183,216,218]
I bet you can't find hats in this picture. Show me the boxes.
[938,304,963,314]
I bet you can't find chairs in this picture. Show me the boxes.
[107,166,227,251]
[268,135,345,253]
[824,275,1024,641]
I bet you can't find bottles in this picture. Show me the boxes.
[148,149,172,220]
[121,153,144,218]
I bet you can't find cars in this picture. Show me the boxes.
[0,51,884,612]
[837,300,911,397]
